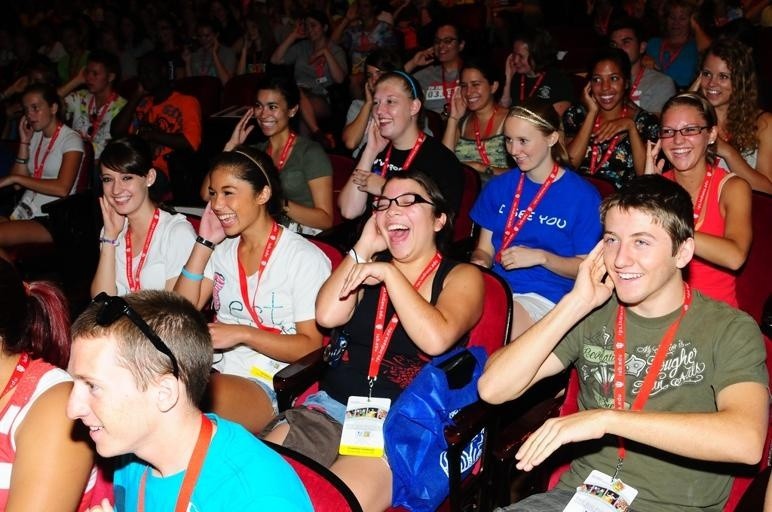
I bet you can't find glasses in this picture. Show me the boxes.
[371,192,436,211]
[657,125,710,139]
[92,290,180,379]
[434,36,457,44]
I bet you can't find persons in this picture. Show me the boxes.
[0,1,771,511]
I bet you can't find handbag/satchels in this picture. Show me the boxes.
[383,346,488,512]
